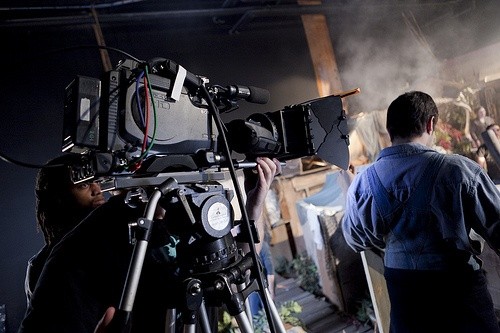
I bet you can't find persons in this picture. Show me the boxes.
[24,156,281,333]
[247,241,275,322]
[340,91,500,333]
